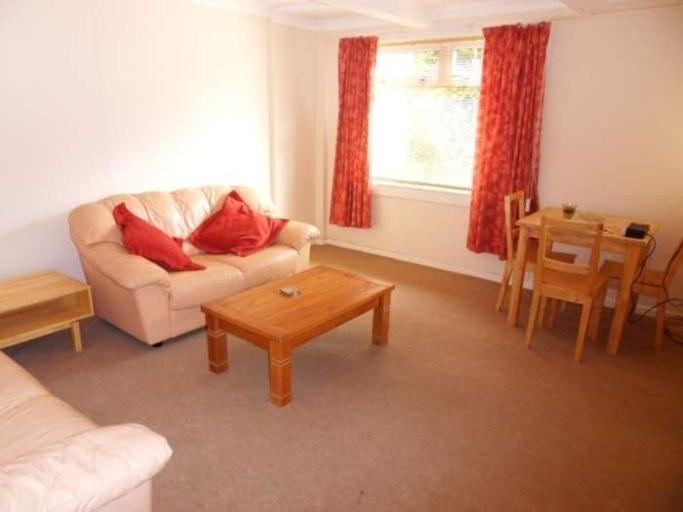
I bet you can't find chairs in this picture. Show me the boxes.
[493,191,683,362]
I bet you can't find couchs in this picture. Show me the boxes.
[66,184,319,347]
[0,349,173,512]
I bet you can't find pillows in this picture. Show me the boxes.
[113,190,289,271]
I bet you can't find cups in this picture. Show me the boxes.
[562,203,577,220]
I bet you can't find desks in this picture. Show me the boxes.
[0,268,96,352]
